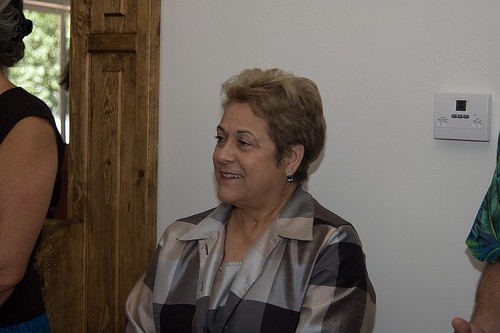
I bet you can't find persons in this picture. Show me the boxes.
[1,0,70,332]
[450,129,500,332]
[124,66,377,332]
